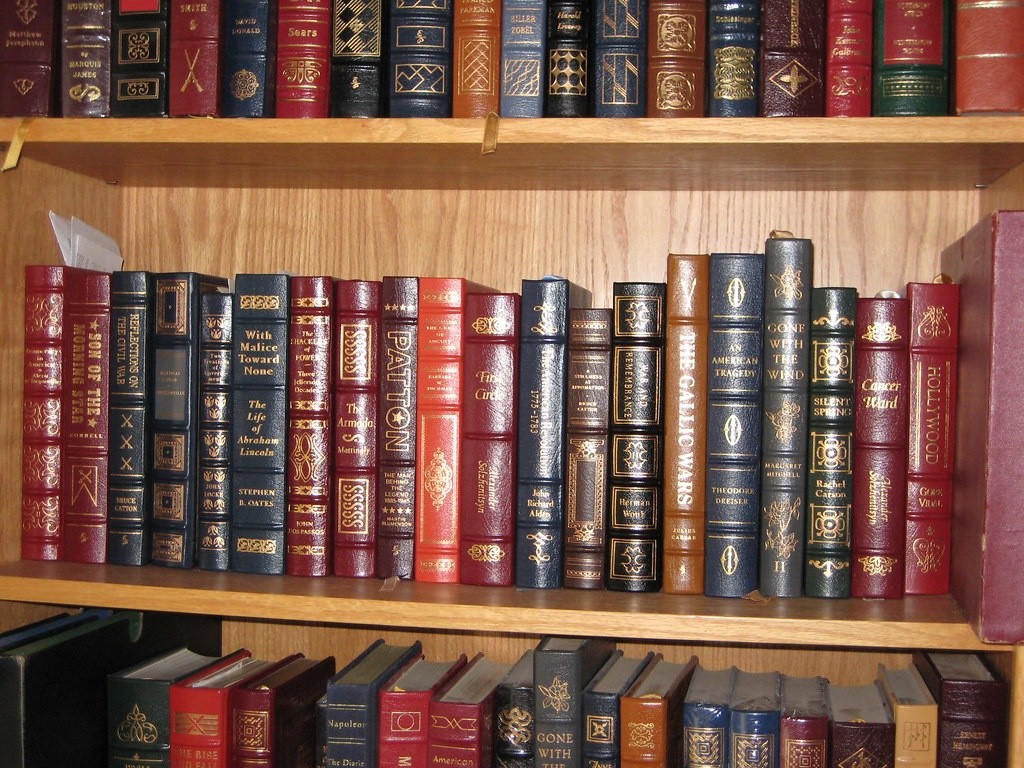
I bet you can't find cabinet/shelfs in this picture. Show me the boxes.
[0,0,1024,768]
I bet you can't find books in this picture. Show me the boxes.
[0,211,1024,768]
[0,0,1024,119]
